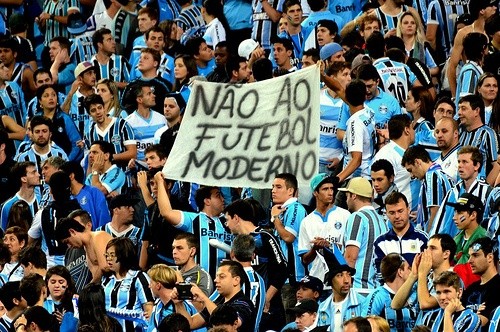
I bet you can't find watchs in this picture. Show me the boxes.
[271,216,277,223]
[92,171,98,175]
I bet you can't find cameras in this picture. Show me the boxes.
[176,282,193,300]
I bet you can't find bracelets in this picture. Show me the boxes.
[50,15,55,20]
[16,323,26,330]
[354,19,357,25]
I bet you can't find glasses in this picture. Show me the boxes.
[391,255,406,282]
[104,253,115,258]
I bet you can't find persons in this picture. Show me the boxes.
[0,0,500,332]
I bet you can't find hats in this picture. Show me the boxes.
[238,39,259,60]
[294,275,323,292]
[66,12,87,34]
[319,42,343,60]
[337,177,373,198]
[322,242,356,286]
[318,20,338,34]
[445,193,484,213]
[310,172,334,193]
[74,61,95,78]
[109,194,141,209]
